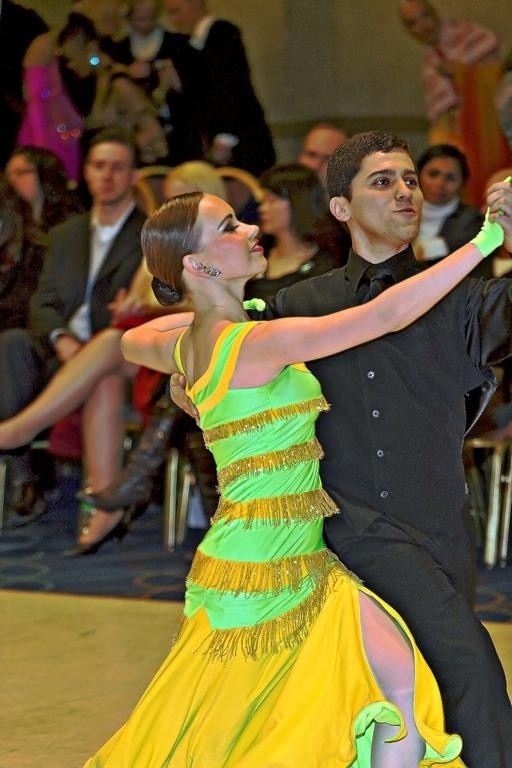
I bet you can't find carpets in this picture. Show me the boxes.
[0,481,512,625]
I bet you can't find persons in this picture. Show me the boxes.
[0,1,276,191]
[164,128,511,768]
[1,125,511,558]
[84,177,511,768]
[400,1,511,209]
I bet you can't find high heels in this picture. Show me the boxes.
[60,509,129,559]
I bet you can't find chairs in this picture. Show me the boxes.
[456,379,511,570]
[0,162,271,552]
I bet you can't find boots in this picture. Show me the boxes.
[74,403,189,525]
